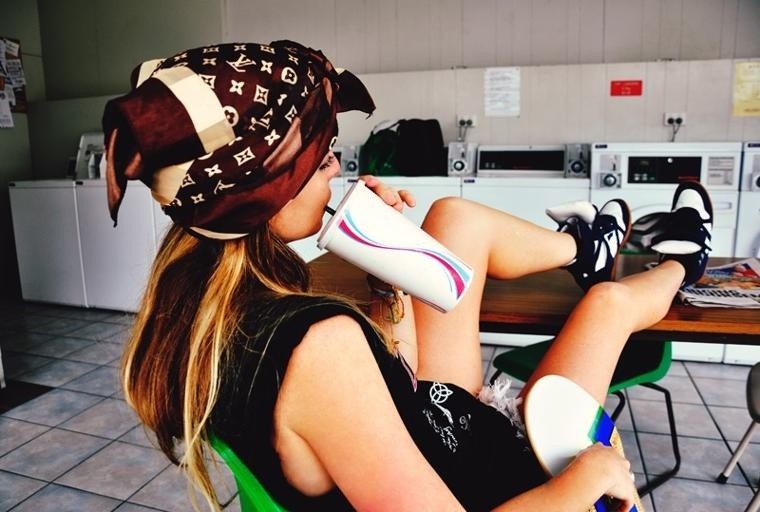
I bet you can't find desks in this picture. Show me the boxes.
[306,251,760,346]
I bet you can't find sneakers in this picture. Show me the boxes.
[545,196,635,293]
[649,181,717,289]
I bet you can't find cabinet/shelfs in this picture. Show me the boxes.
[153,199,174,248]
[344,185,461,229]
[722,191,760,366]
[590,189,739,365]
[284,186,344,264]
[73,187,155,314]
[463,187,590,346]
[9,187,87,309]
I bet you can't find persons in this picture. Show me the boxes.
[102,39,715,512]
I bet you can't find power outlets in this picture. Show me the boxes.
[663,113,687,125]
[457,114,476,127]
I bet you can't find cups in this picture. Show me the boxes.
[318,178,473,315]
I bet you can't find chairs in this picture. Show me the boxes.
[718,361,760,512]
[489,248,680,499]
[209,431,289,511]
